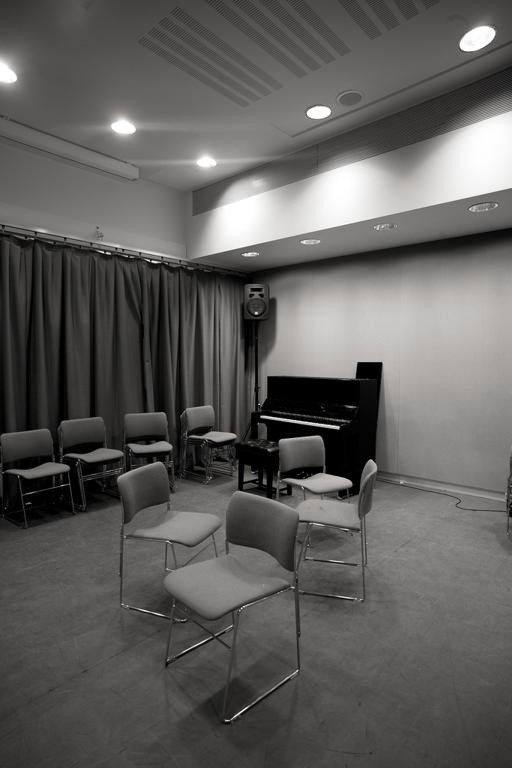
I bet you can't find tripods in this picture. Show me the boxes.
[232,322,266,463]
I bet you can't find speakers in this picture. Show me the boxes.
[243,284,271,320]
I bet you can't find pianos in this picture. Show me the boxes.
[252,376,379,499]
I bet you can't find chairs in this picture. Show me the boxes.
[289,458,382,604]
[113,456,225,624]
[161,490,307,726]
[275,433,354,506]
[1,404,238,529]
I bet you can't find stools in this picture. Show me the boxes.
[235,437,293,500]
